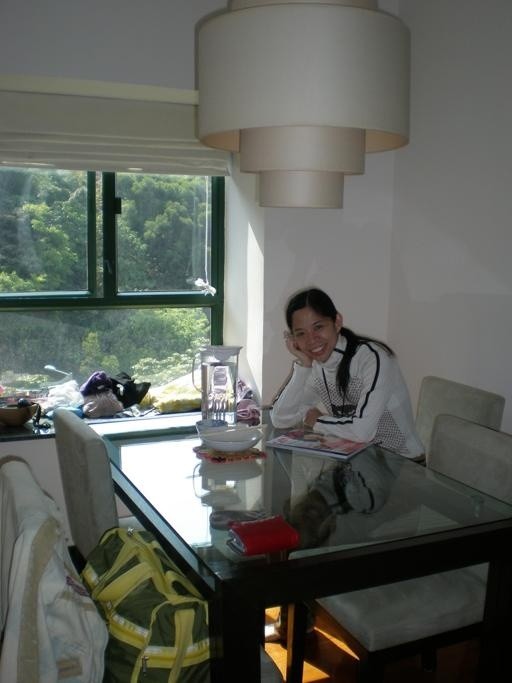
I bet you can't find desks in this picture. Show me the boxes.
[100,416,512,683]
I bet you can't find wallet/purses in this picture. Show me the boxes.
[226,514,300,556]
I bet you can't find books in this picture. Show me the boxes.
[261,428,389,461]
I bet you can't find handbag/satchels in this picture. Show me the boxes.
[78,526,212,682]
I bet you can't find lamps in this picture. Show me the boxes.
[193,0,411,208]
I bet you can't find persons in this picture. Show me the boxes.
[282,441,406,547]
[257,286,428,644]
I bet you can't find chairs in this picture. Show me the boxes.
[52,406,144,562]
[414,375,506,466]
[1,456,284,683]
[286,416,512,683]
